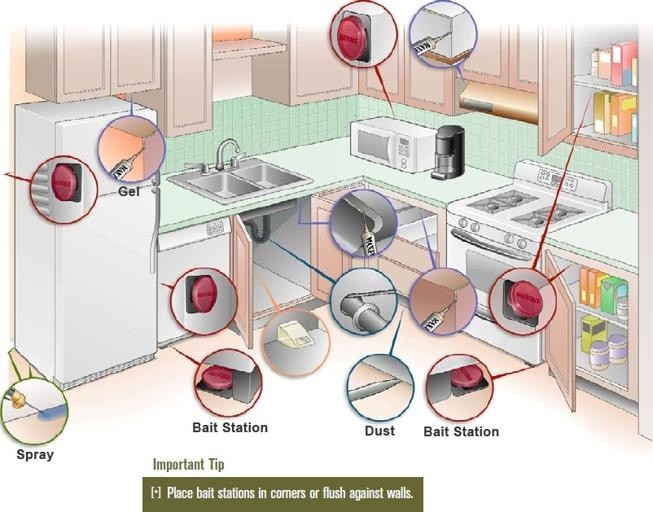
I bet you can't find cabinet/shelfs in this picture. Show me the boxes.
[357,12,476,117]
[455,9,637,160]
[26,23,160,104]
[540,243,640,414]
[323,183,447,297]
[125,21,214,139]
[233,198,357,349]
[253,21,360,108]
